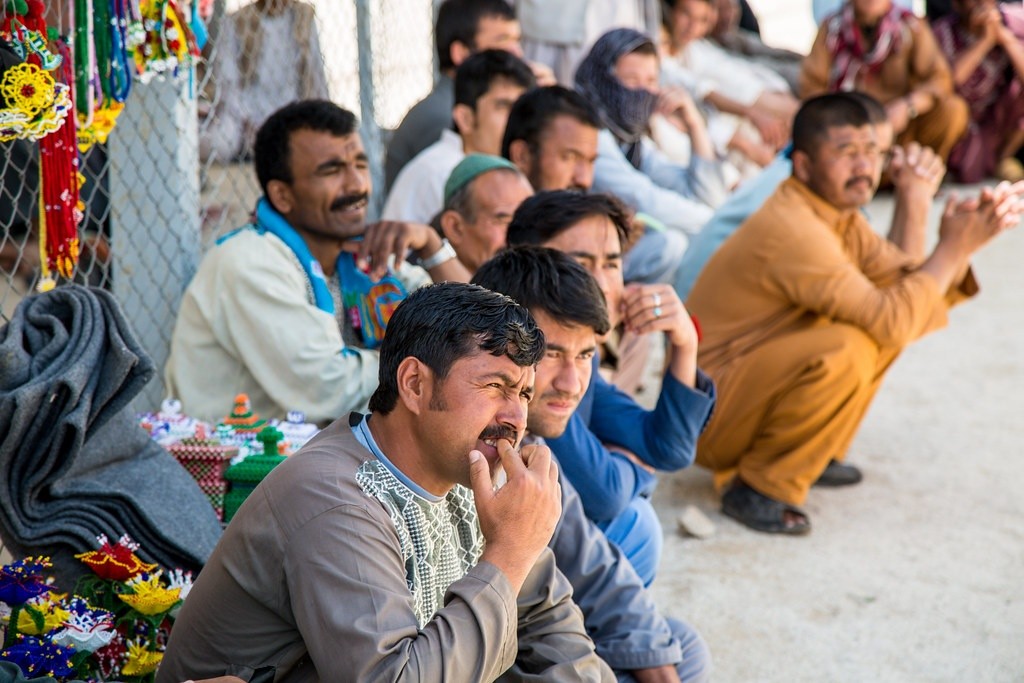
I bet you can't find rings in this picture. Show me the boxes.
[653,296,661,306]
[653,308,661,318]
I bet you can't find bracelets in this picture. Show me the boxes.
[903,95,918,119]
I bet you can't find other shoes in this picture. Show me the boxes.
[816,457,862,485]
[721,469,812,534]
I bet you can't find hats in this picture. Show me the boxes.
[429,152,517,239]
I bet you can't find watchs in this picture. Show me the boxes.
[420,238,457,272]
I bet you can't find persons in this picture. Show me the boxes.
[469,246,710,683]
[682,91,1024,534]
[162,99,473,430]
[156,281,619,683]
[380,0,1024,303]
[505,190,714,589]
